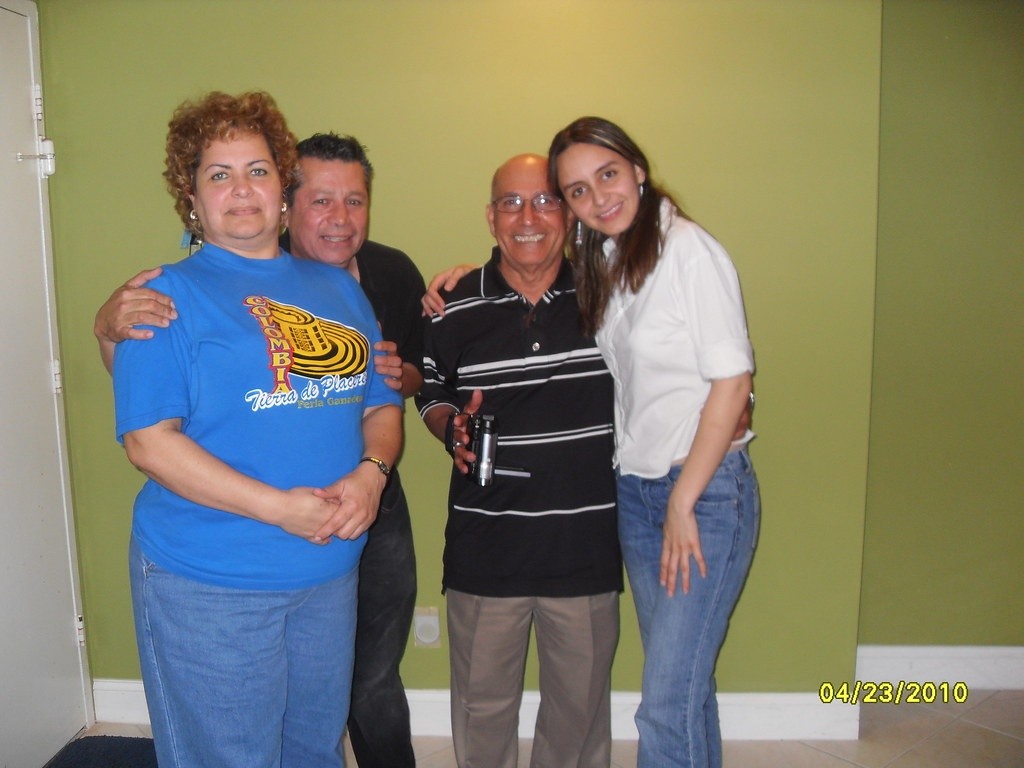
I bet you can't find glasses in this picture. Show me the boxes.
[492,195,562,214]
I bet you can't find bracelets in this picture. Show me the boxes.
[747,391,756,412]
[359,456,392,479]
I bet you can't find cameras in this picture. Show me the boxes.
[465,414,498,486]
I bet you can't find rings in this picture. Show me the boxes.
[453,442,465,453]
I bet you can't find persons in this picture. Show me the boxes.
[92,133,427,768]
[421,118,763,768]
[114,92,405,768]
